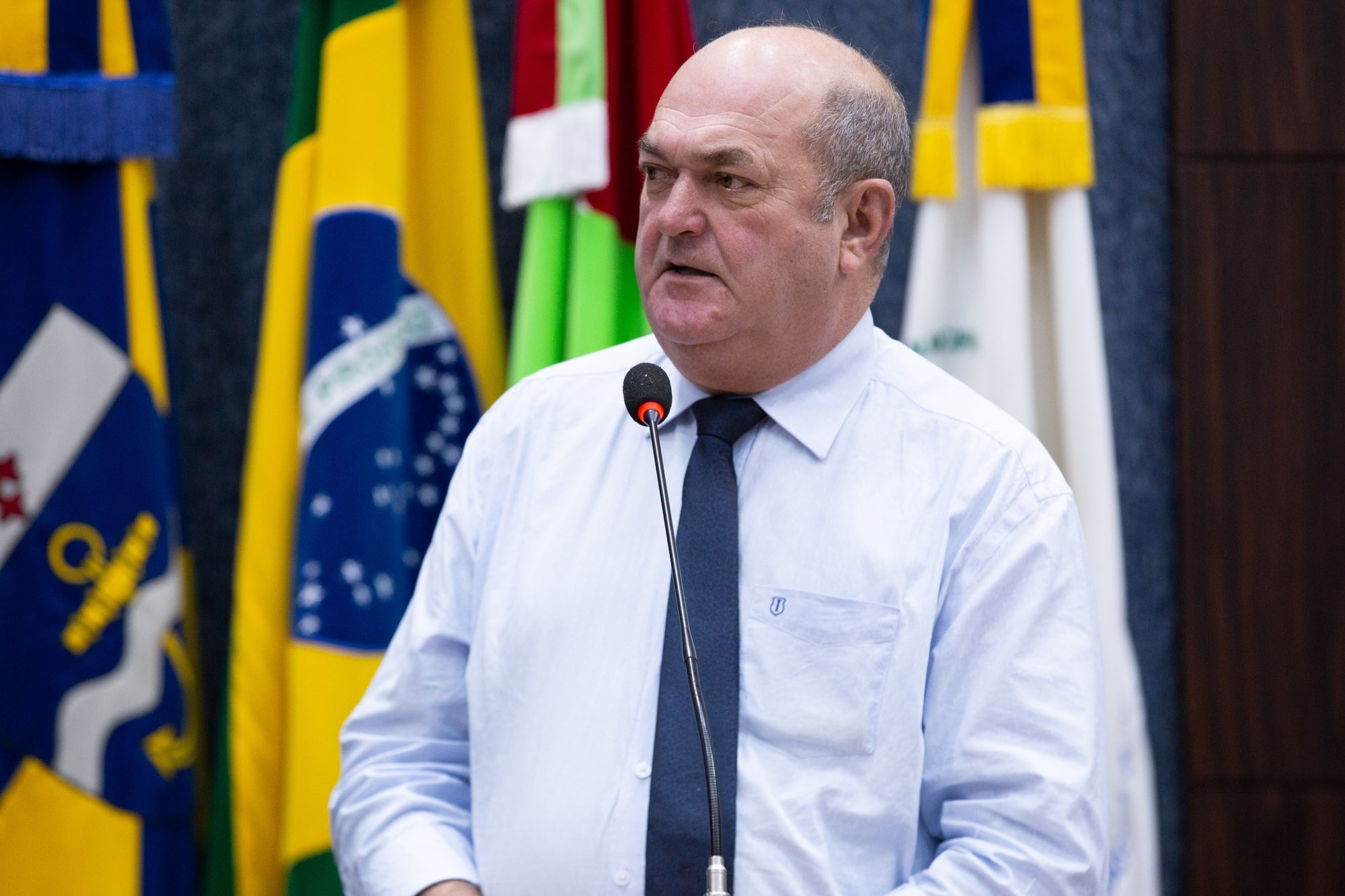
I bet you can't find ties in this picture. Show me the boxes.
[644,396,764,896]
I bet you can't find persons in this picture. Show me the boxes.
[328,25,1112,896]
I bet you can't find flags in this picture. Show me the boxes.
[228,0,1170,896]
[0,0,207,896]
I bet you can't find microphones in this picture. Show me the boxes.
[622,363,729,895]
